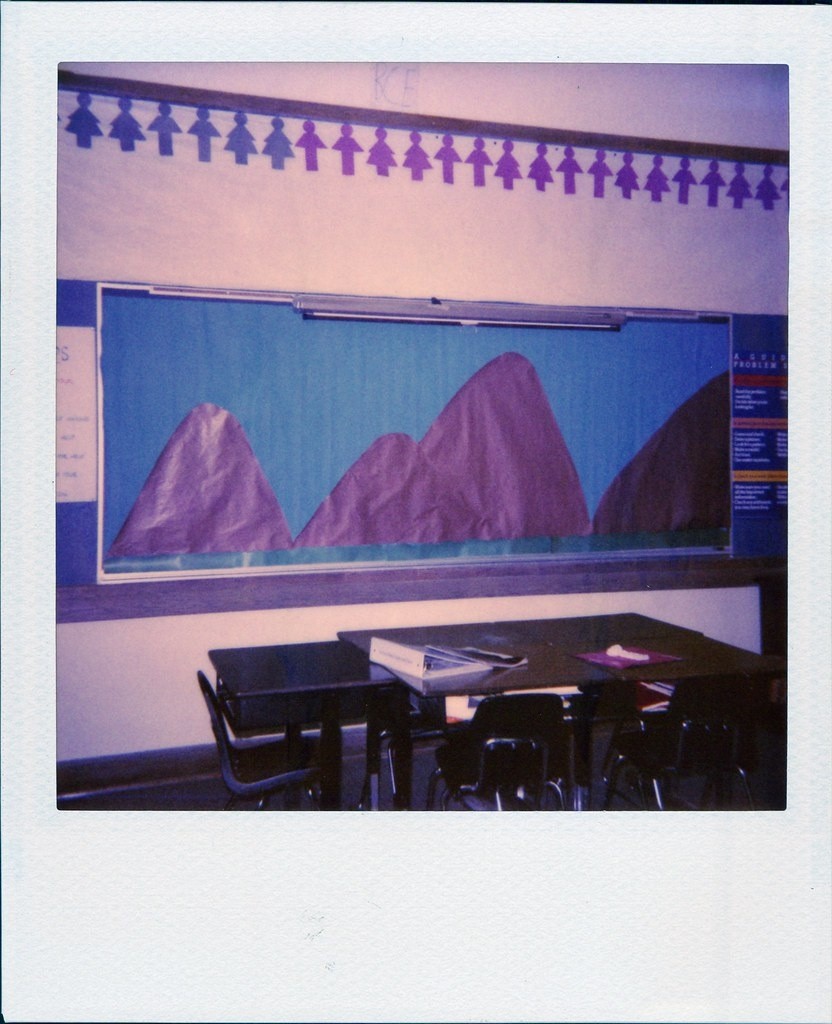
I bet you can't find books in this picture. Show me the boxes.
[636,681,675,713]
[426,643,529,669]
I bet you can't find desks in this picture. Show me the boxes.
[209,642,413,811]
[338,613,784,811]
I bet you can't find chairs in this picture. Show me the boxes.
[602,672,756,810]
[429,694,573,811]
[196,670,343,810]
